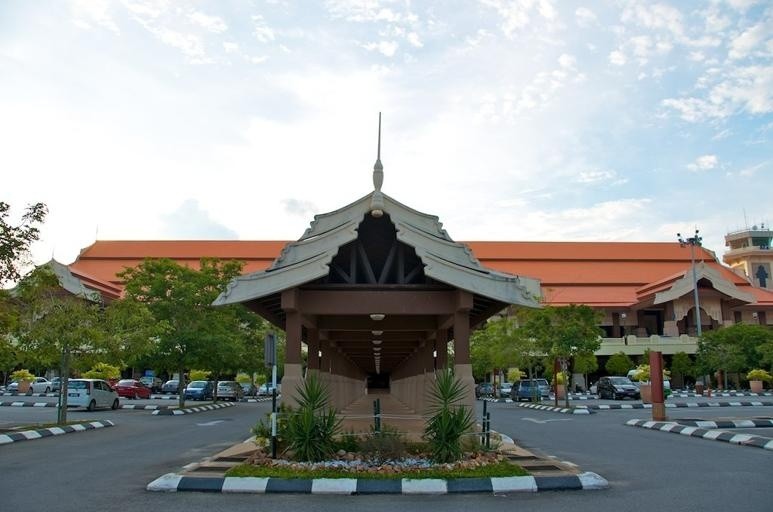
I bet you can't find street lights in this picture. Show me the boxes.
[752,312,759,324]
[621,312,627,345]
[677,230,707,387]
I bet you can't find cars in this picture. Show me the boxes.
[475,378,550,402]
[589,375,641,400]
[8,376,282,412]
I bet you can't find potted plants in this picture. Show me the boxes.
[634,364,665,404]
[747,369,773,393]
[9,369,36,393]
[551,372,569,400]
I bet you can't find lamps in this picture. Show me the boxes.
[370,314,385,375]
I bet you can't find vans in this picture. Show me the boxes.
[627,369,672,400]
